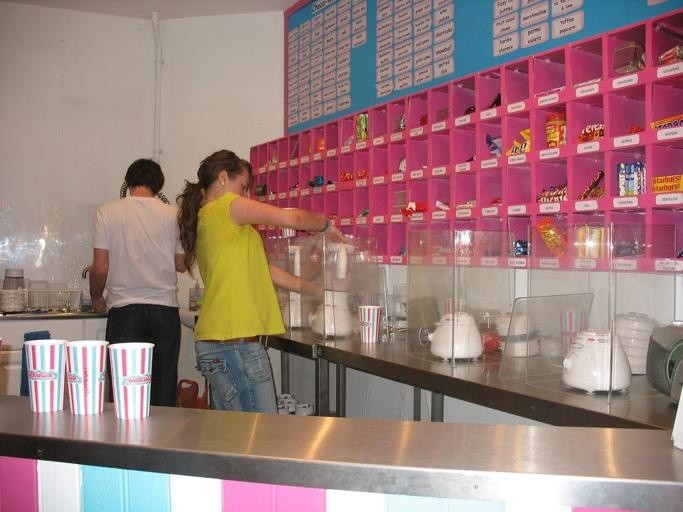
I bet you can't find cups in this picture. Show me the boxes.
[23,339,67,413]
[107,341,155,421]
[65,340,109,416]
[27,279,72,311]
[359,305,381,344]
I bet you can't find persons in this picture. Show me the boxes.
[87,158,190,405]
[178,152,348,415]
[31,226,50,268]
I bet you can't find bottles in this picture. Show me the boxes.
[4,269,25,290]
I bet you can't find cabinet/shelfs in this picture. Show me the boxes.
[476,9,682,271]
[252,110,371,261]
[373,75,476,267]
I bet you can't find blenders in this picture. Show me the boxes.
[562,328,632,397]
[427,311,483,363]
[282,243,315,328]
[306,242,359,337]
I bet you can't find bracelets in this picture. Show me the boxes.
[315,213,335,237]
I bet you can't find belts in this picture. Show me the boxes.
[224,336,269,348]
